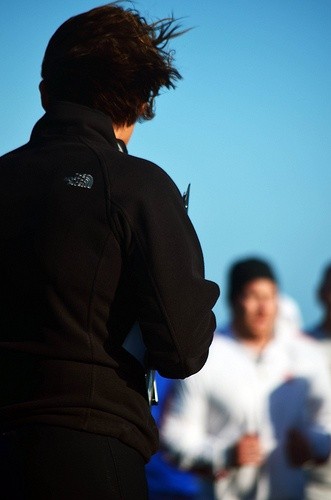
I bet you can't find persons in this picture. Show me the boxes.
[144,256,331,500]
[0,0,221,500]
